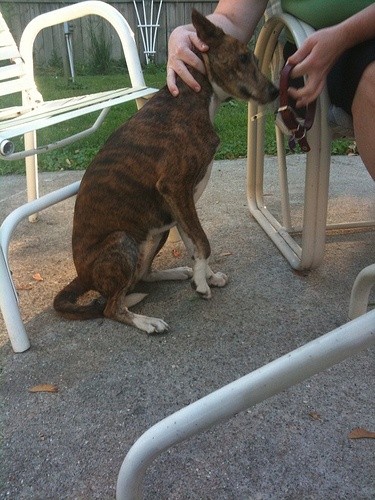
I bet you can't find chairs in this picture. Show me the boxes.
[0,0,159,353]
[116,13,375,500]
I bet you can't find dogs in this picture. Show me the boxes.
[53,9,281,336]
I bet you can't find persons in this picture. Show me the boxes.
[167,0,375,181]
